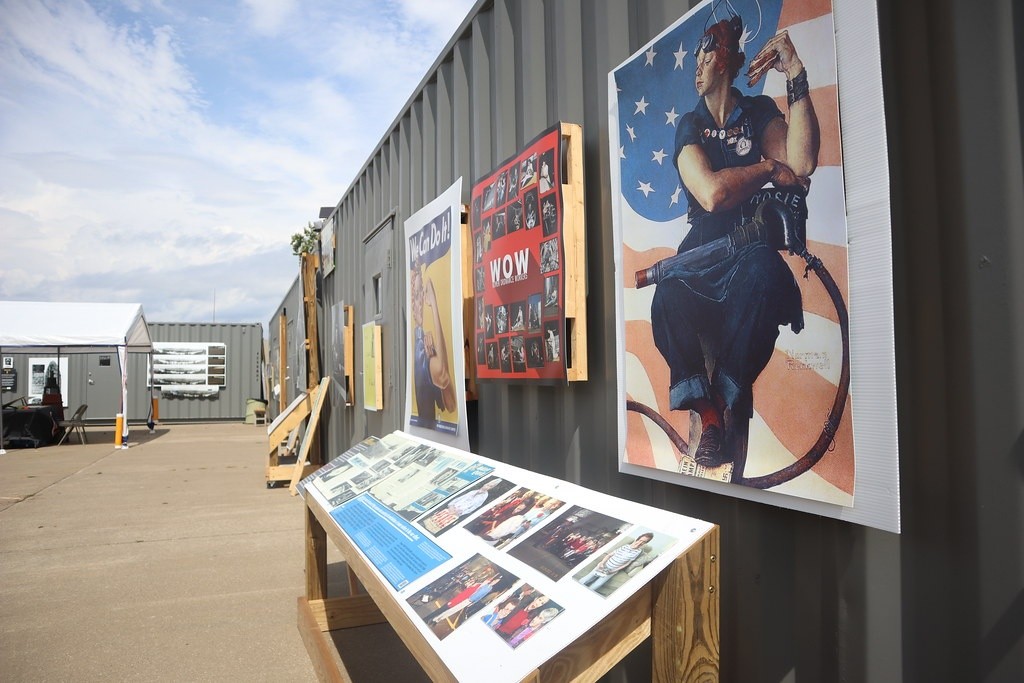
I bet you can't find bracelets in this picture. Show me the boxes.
[786,67,809,103]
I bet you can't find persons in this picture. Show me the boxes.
[319,435,654,649]
[476,158,557,371]
[410,259,458,422]
[651,17,822,465]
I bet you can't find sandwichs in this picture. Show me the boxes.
[745,50,778,88]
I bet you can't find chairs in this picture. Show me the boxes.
[58,404,88,446]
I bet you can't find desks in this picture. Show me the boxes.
[0,403,69,449]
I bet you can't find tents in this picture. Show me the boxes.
[0,301,155,450]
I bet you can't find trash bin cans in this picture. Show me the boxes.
[245,398,268,424]
